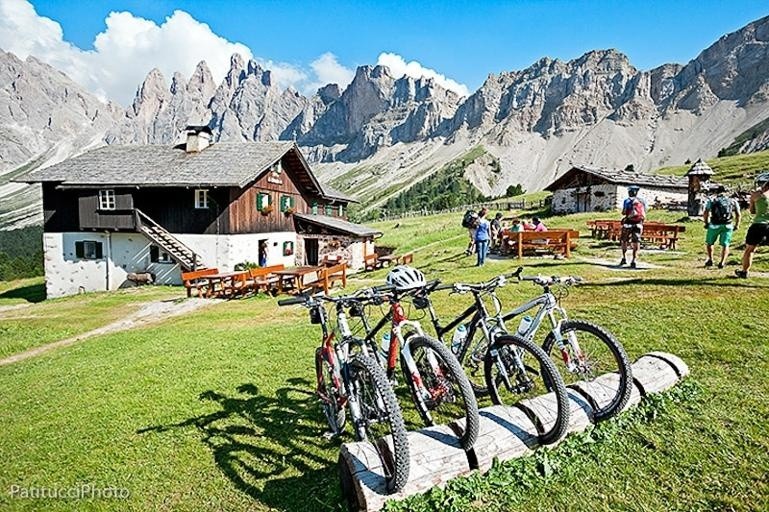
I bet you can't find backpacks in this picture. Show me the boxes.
[462,208,478,228]
[626,198,644,223]
[710,194,733,224]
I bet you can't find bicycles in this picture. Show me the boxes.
[342,272,482,451]
[452,272,636,424]
[393,264,572,446]
[277,282,413,499]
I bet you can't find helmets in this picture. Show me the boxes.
[754,173,768,182]
[628,186,640,192]
[384,263,426,294]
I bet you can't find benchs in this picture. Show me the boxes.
[506,218,686,260]
[179,251,414,300]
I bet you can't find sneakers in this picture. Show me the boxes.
[735,269,747,279]
[706,261,712,266]
[631,263,635,268]
[621,259,626,265]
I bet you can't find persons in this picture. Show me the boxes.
[464,207,548,267]
[703,185,741,269]
[620,185,647,268]
[735,174,769,278]
